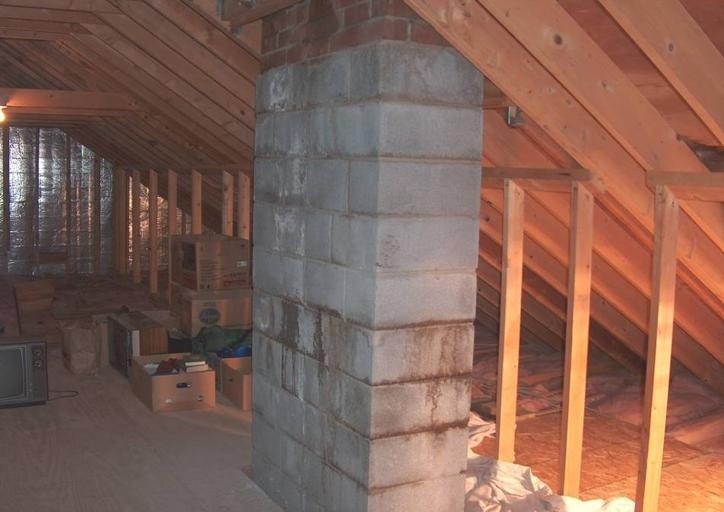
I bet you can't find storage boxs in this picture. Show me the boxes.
[128,233,252,415]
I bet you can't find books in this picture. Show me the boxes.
[143,355,209,375]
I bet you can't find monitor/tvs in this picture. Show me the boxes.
[0,335,48,409]
